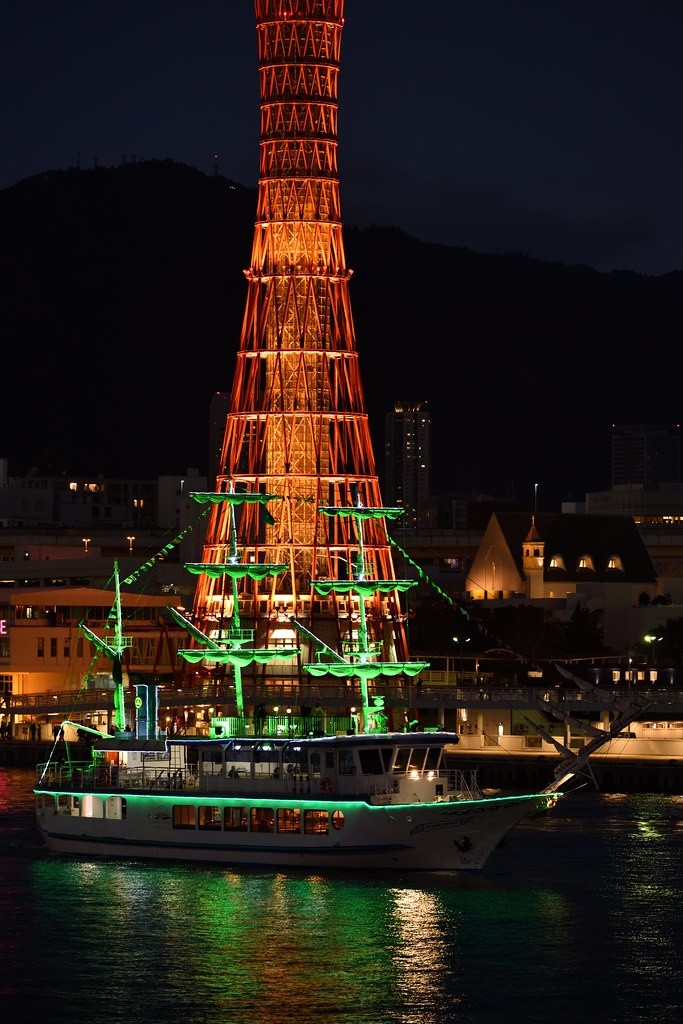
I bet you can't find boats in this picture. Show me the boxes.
[31,491,640,879]
